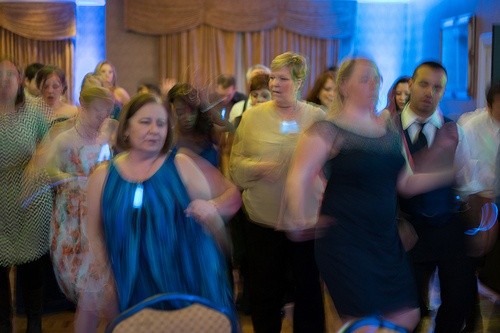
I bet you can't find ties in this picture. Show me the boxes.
[414,122,429,158]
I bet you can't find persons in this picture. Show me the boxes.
[0,51,500,333]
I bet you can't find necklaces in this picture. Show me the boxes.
[74,124,100,139]
[126,153,162,208]
